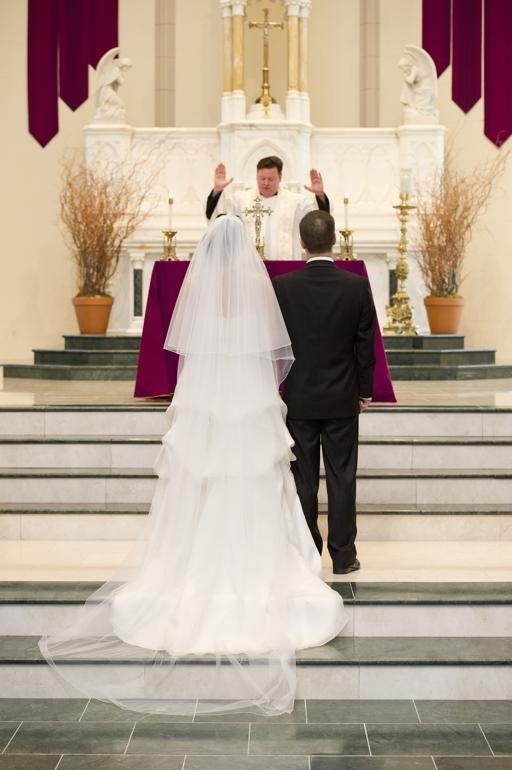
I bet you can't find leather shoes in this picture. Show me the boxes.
[334,558,360,574]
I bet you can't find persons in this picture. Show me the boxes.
[204,156,330,260]
[34,213,351,717]
[268,209,376,575]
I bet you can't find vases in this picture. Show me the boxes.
[423,294,465,334]
[72,289,116,336]
